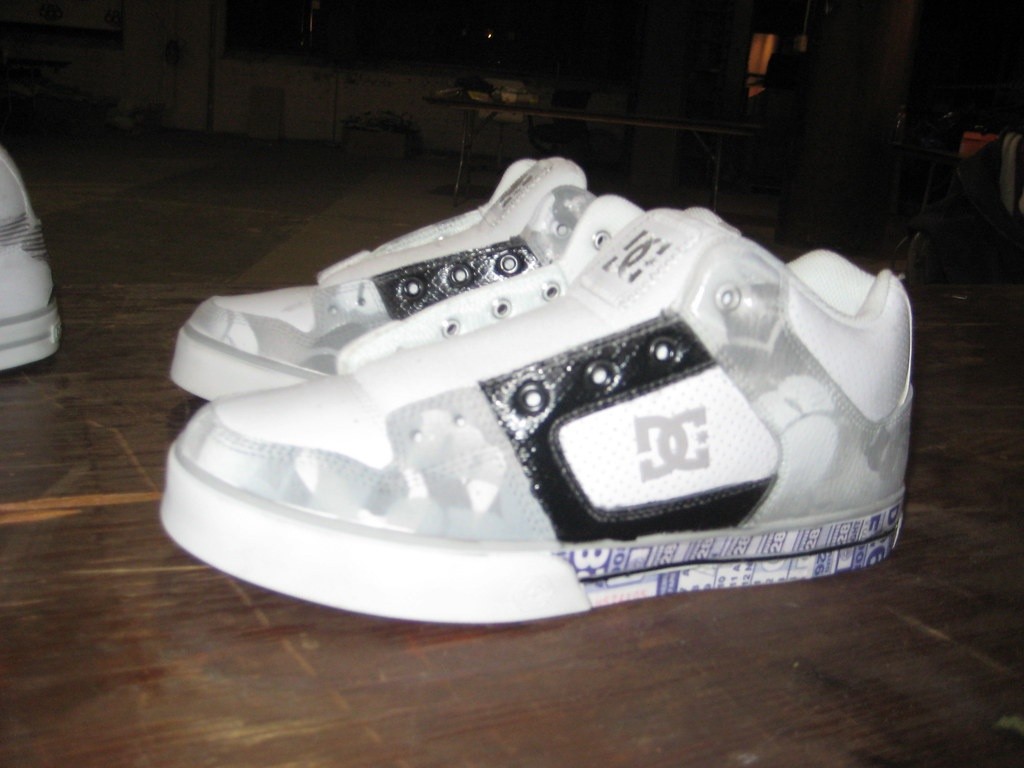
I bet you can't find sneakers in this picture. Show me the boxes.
[0,148,61,372]
[168,156,647,400]
[161,204,914,626]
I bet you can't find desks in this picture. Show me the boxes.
[903,143,973,215]
[422,95,763,211]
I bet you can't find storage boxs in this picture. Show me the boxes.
[341,129,419,160]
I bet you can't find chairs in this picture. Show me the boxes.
[527,90,593,164]
